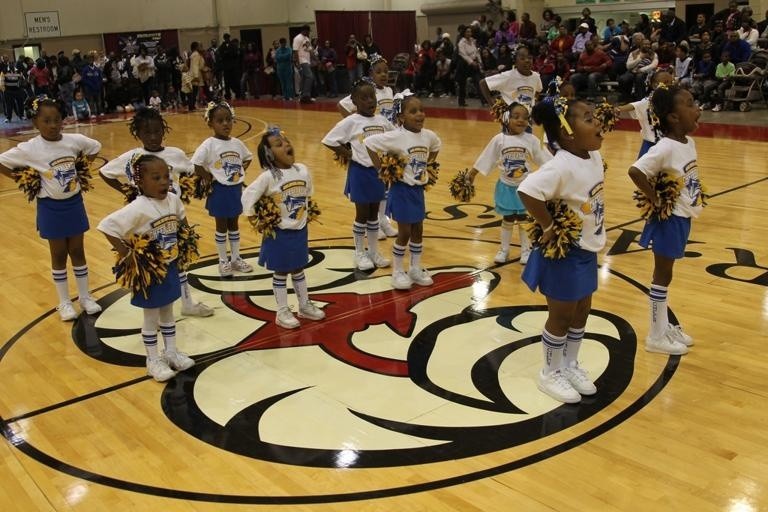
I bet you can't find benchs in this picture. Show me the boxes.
[564,68,651,99]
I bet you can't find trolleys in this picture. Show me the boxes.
[474,58,501,106]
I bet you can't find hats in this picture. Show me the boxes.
[470,21,480,27]
[579,22,589,29]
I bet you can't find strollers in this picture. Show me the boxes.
[388,51,409,95]
[722,50,768,111]
[198,64,231,109]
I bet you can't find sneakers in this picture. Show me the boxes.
[181,302,214,317]
[59,299,78,321]
[520,252,530,264]
[163,348,195,371]
[231,258,253,273]
[276,305,300,330]
[146,356,176,382]
[218,258,233,277]
[712,104,721,111]
[79,294,101,315]
[537,365,595,403]
[645,324,693,355]
[699,103,706,111]
[494,247,510,263]
[354,222,433,291]
[298,303,325,321]
